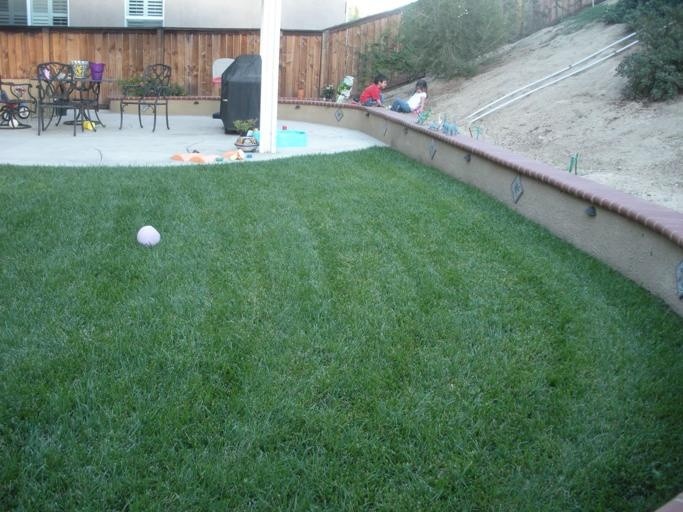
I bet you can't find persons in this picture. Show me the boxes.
[359,73,388,107]
[390,79,429,113]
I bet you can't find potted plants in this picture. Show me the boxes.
[232,118,259,152]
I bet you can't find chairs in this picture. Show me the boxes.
[119,63,172,132]
[1,61,105,136]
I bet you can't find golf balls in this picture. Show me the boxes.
[138,225,161,246]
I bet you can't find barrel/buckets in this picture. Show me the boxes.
[89,62,106,80]
[71,60,89,79]
